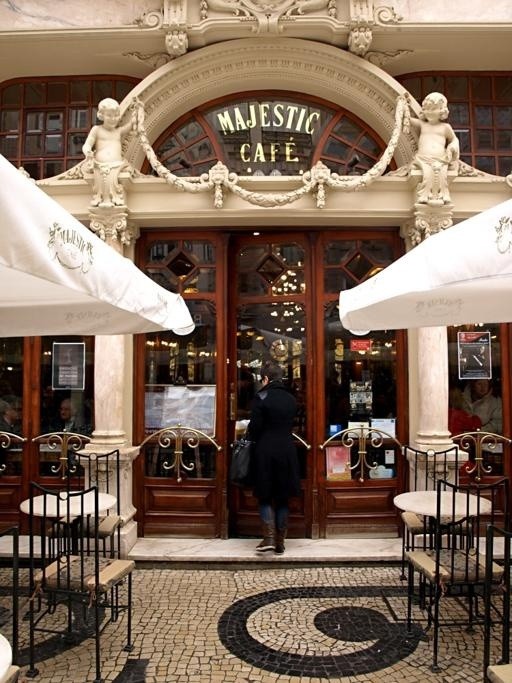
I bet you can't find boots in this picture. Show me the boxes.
[274,526,288,554]
[255,521,277,552]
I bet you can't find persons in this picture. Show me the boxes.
[242,363,303,555]
[448,368,504,483]
[395,92,461,203]
[1,384,94,476]
[81,98,138,205]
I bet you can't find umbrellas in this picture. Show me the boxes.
[0,150,194,339]
[337,199,512,337]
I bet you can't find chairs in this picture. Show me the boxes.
[24,449,136,683]
[402,443,510,673]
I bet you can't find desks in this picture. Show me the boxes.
[19,487,115,647]
[392,491,489,635]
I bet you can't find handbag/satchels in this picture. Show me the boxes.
[229,439,252,486]
